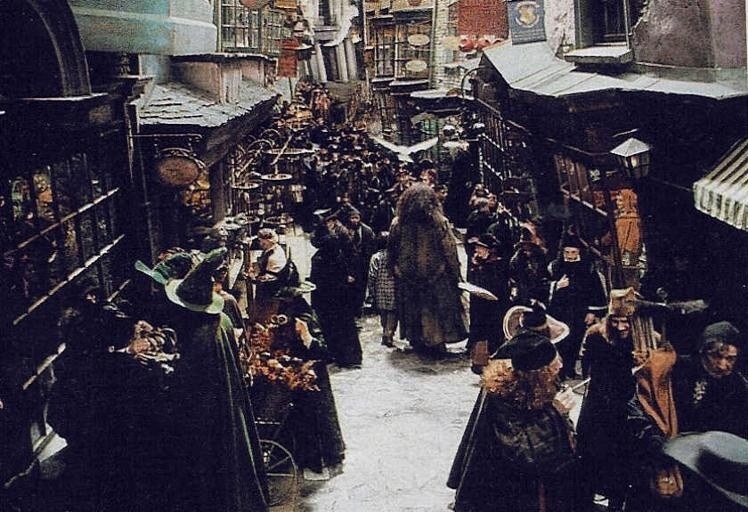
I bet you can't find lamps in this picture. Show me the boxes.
[610,126,655,182]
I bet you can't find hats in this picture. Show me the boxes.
[605,286,638,319]
[689,319,746,354]
[559,227,586,250]
[163,246,231,317]
[500,299,571,347]
[133,250,194,287]
[487,329,559,375]
[513,227,538,251]
[464,229,498,249]
[250,198,381,302]
